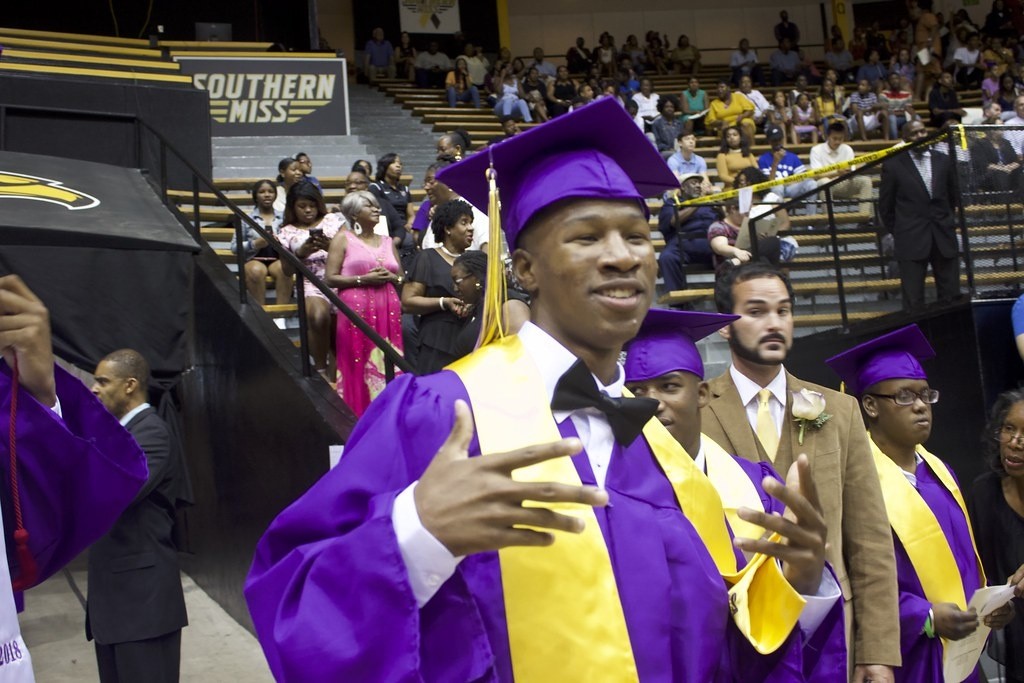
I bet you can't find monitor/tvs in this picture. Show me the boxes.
[195,22,232,42]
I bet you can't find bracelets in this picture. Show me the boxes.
[356,275,361,286]
[397,276,403,287]
[439,297,446,311]
[928,607,935,636]
[295,250,305,259]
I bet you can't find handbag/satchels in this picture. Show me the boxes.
[251,241,282,265]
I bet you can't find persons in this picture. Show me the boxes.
[85,348,188,683]
[231,129,1015,421]
[963,388,1024,683]
[242,98,827,683]
[363,0,1024,146]
[619,308,847,683]
[824,325,1015,683]
[694,257,902,683]
[879,119,965,310]
[0,274,151,683]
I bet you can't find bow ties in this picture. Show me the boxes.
[550,358,660,449]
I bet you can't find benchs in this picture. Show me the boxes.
[1,29,1024,325]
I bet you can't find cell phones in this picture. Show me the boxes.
[265,225,273,232]
[309,228,324,238]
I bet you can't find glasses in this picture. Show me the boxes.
[993,426,1024,449]
[862,389,939,406]
[298,159,310,165]
[448,273,472,289]
[344,180,367,186]
[424,176,435,184]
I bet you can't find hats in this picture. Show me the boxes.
[679,173,704,186]
[435,98,682,354]
[622,308,742,384]
[767,126,783,140]
[824,324,936,394]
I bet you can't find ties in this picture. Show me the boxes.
[756,388,779,463]
[917,154,932,197]
